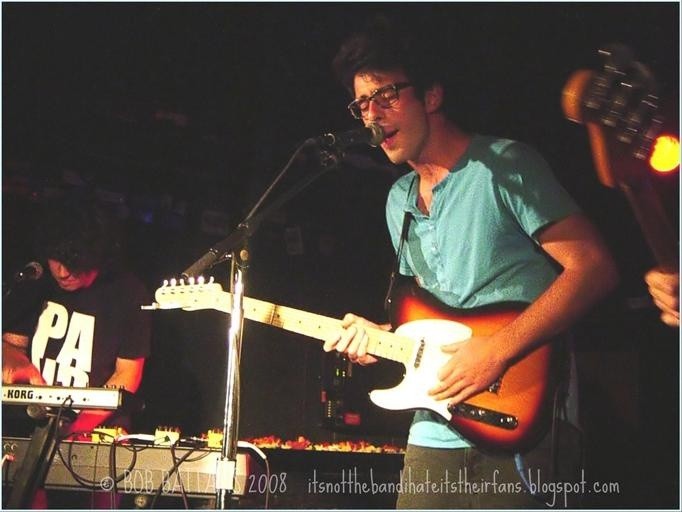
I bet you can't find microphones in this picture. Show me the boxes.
[3,262,46,286]
[307,123,384,148]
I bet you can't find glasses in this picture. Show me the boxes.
[344,83,414,120]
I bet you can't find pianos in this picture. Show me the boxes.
[3,437,311,509]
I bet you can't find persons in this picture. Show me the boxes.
[3,226,152,507]
[320,33,619,508]
[643,262,680,328]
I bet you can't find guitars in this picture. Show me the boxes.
[153,276,556,453]
[563,41,681,273]
